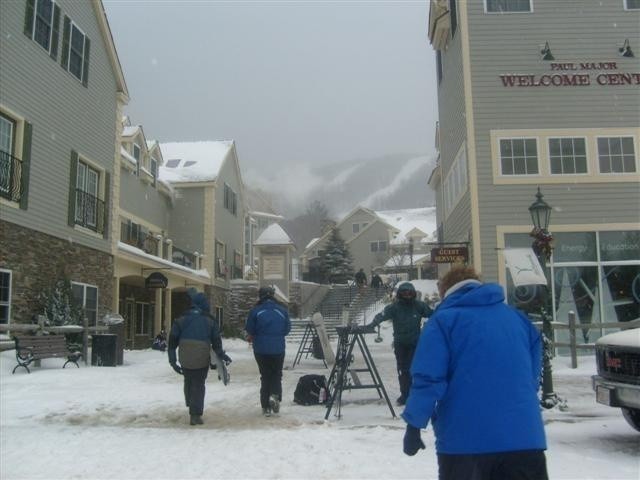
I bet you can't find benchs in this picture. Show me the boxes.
[12,334,84,377]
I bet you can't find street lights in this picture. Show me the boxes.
[528,187,567,414]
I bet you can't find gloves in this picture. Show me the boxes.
[171,361,184,374]
[404,423,425,456]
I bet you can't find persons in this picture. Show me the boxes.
[370,273,383,299]
[167,293,223,426]
[401,266,548,480]
[367,283,434,404]
[355,268,367,295]
[245,286,291,418]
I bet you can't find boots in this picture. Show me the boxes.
[189,406,203,425]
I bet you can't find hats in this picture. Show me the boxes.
[260,287,275,299]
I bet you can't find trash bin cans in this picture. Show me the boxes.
[91,314,125,366]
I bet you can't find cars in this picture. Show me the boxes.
[590,327,640,435]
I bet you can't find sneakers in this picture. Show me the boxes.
[262,394,280,417]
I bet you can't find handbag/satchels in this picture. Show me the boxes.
[293,374,331,406]
